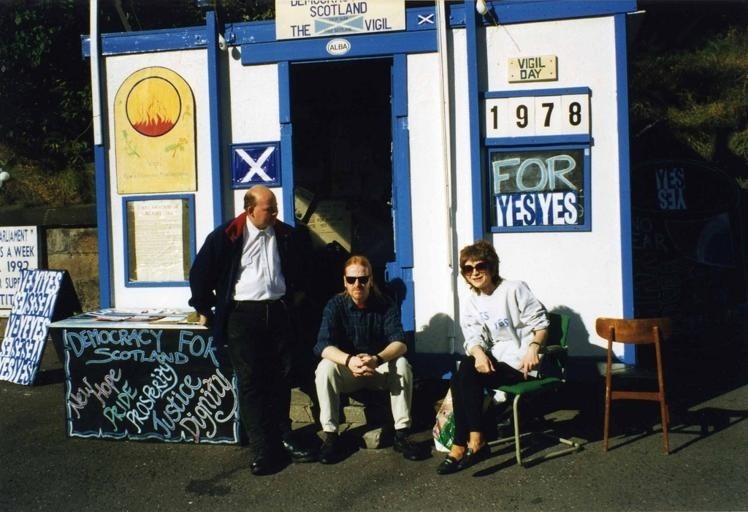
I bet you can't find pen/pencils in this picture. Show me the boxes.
[177,322,202,325]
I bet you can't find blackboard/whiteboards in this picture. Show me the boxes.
[63,328,243,445]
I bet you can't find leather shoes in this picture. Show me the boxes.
[437,441,492,475]
[394,437,426,462]
[250,436,312,476]
[319,443,334,465]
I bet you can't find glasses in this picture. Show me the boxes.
[344,274,371,286]
[461,262,490,277]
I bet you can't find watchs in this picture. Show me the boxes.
[377,355,385,366]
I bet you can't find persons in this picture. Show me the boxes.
[437,240,549,476]
[189,187,307,474]
[310,256,429,463]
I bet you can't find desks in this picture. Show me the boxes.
[46,308,241,447]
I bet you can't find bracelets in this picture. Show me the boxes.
[529,342,542,347]
[346,353,353,367]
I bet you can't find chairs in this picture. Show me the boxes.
[489,313,676,466]
